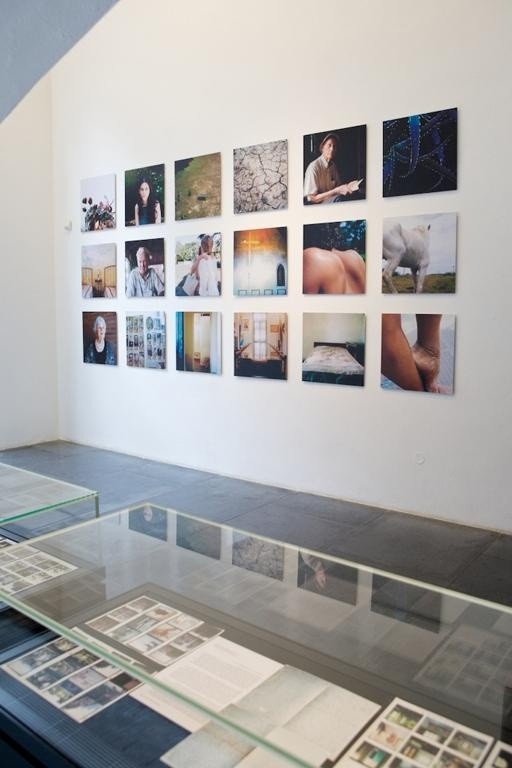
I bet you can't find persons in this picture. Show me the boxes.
[134,175,162,225]
[303,132,365,204]
[84,314,114,365]
[190,234,219,296]
[303,221,367,294]
[125,246,165,296]
[382,312,453,395]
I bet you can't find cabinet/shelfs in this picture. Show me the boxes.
[1,502,511,768]
[1,462,102,651]
